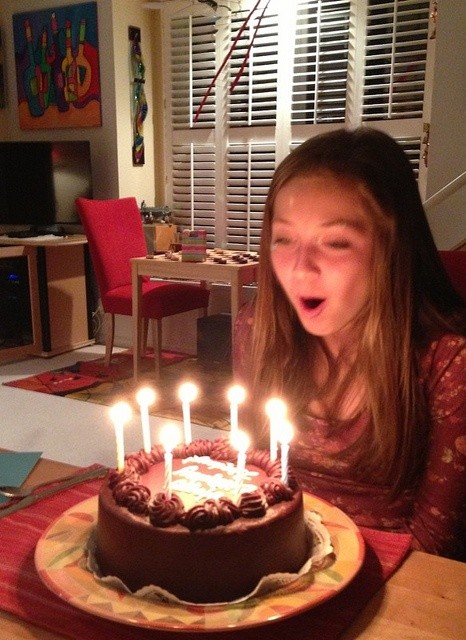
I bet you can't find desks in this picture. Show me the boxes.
[0,448,465,638]
[129,248,261,386]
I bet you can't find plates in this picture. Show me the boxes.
[34,490,367,634]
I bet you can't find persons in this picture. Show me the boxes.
[230,128,466,557]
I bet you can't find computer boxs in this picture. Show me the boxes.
[1,248,50,352]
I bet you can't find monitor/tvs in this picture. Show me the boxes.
[1,141,95,240]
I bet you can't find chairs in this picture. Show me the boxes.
[75,196,209,380]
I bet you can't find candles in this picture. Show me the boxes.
[158,424,183,500]
[276,420,294,483]
[178,383,199,443]
[264,399,286,463]
[109,403,133,472]
[230,434,252,497]
[224,388,247,436]
[134,387,156,452]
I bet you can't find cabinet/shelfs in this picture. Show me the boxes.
[1,234,95,364]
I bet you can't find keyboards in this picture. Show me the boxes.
[1,233,68,242]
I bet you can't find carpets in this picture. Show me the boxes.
[2,347,196,396]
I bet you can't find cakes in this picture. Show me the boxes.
[96,440,307,605]
